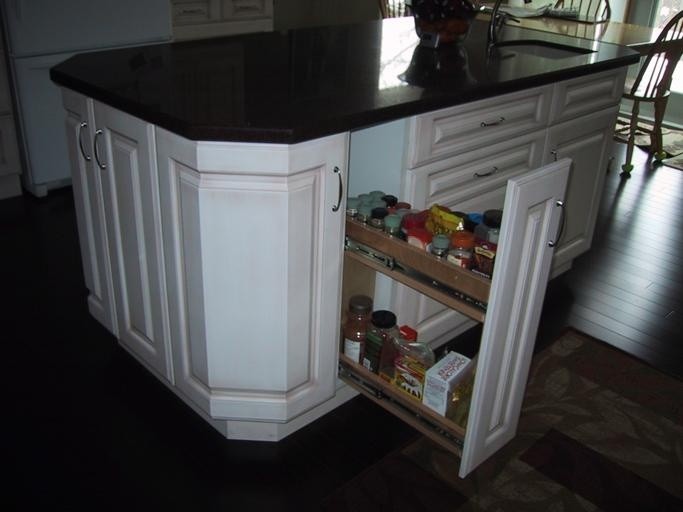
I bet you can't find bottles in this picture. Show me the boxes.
[343,295,373,364]
[384,215,401,235]
[370,190,384,198]
[346,197,359,217]
[396,202,410,209]
[369,208,386,229]
[383,195,397,207]
[371,199,387,208]
[396,208,410,218]
[359,194,373,202]
[363,311,399,372]
[358,202,373,222]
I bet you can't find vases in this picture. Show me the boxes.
[404,3,491,49]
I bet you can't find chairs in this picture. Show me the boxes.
[608,9,683,176]
[549,1,616,23]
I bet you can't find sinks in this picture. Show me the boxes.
[500,40,597,59]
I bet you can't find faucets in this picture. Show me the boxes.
[487,0,531,57]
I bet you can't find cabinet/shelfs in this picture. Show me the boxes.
[386,83,553,356]
[540,65,632,284]
[62,74,175,394]
[156,128,347,442]
[338,120,571,484]
